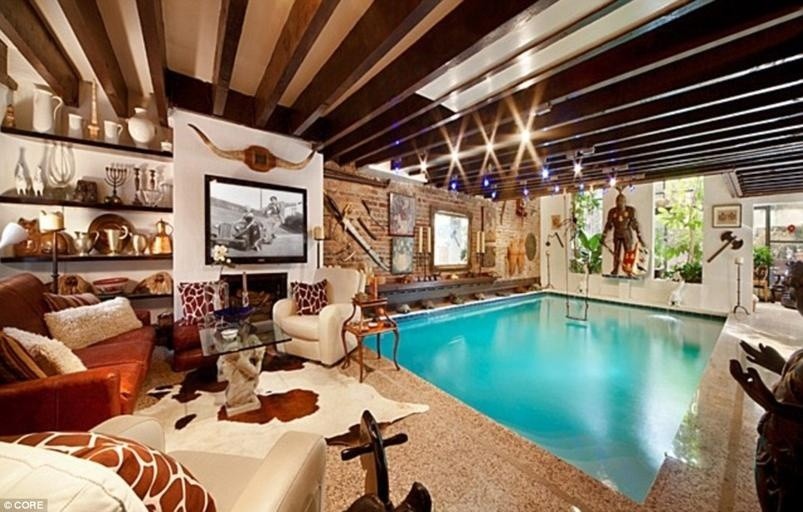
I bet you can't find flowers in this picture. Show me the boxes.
[210,245,235,280]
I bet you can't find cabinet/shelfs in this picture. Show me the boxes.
[340,294,400,383]
[0,124,174,351]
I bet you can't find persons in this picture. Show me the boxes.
[262,196,302,244]
[596,194,644,275]
[235,212,261,252]
[731,261,800,512]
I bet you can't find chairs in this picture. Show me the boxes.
[0,414,326,511]
[271,268,367,369]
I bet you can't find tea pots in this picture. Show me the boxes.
[72,217,174,257]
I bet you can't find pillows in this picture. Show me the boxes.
[176,280,230,327]
[290,278,330,316]
[1,291,143,378]
[11,432,219,512]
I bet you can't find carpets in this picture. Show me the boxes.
[131,355,431,460]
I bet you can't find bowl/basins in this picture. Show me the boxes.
[93,277,129,295]
[220,328,239,341]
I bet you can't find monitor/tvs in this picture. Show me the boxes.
[204,175,307,265]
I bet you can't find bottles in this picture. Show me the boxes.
[160,139,173,154]
[126,107,156,149]
[368,318,378,329]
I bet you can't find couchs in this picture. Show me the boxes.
[0,272,160,432]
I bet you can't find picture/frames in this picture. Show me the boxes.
[711,204,742,228]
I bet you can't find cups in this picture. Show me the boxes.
[160,183,173,207]
[31,87,124,146]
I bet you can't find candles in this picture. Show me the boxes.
[417,226,422,252]
[427,227,431,252]
[475,231,485,253]
